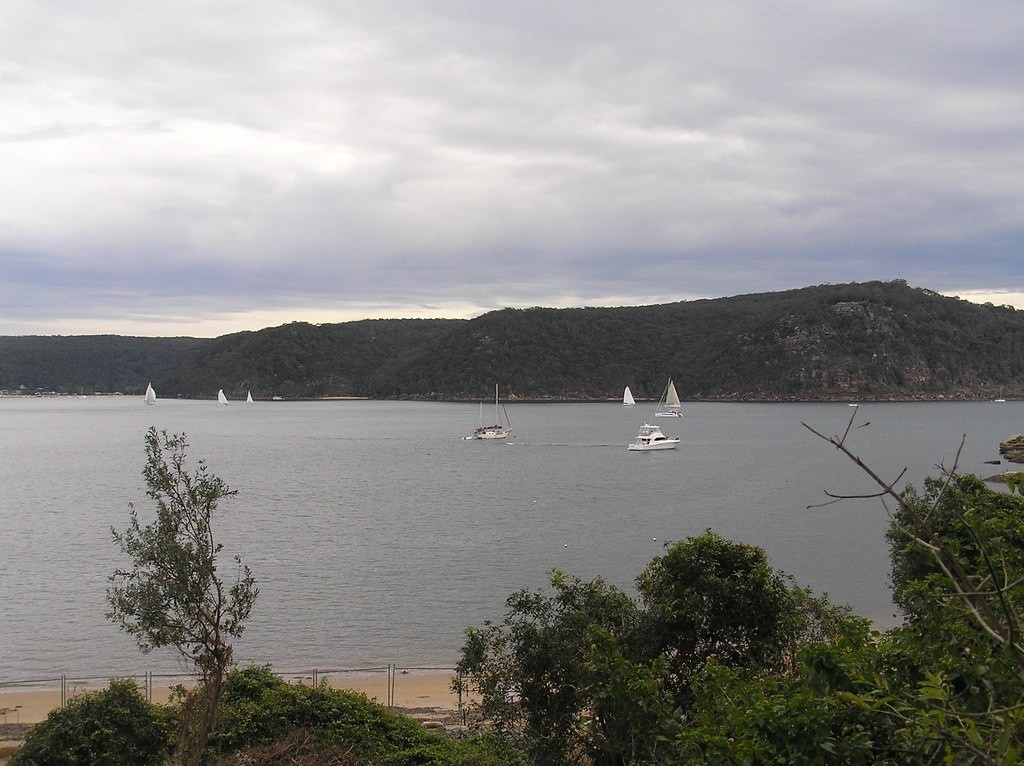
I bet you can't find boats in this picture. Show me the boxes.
[627,424,681,451]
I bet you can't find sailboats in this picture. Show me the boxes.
[664,381,681,407]
[145,382,157,405]
[623,386,635,406]
[216,389,229,406]
[247,390,254,403]
[654,377,684,418]
[473,383,512,440]
